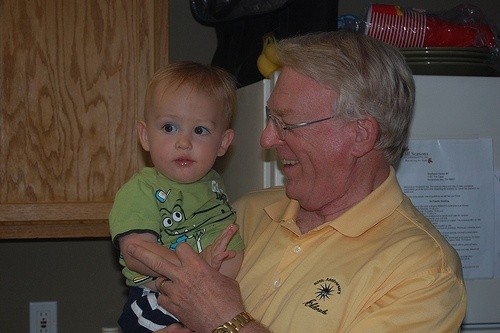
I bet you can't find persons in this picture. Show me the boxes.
[108,61,244,333]
[126,30,467,333]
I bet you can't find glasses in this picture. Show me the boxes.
[265,105,336,141]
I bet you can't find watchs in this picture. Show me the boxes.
[212,312,256,333]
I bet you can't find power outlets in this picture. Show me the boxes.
[29,302,57,333]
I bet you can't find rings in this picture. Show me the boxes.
[160,279,170,290]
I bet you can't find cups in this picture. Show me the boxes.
[365,3,494,50]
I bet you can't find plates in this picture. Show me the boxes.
[401,53,498,74]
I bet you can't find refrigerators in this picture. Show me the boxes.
[214,74,500,333]
